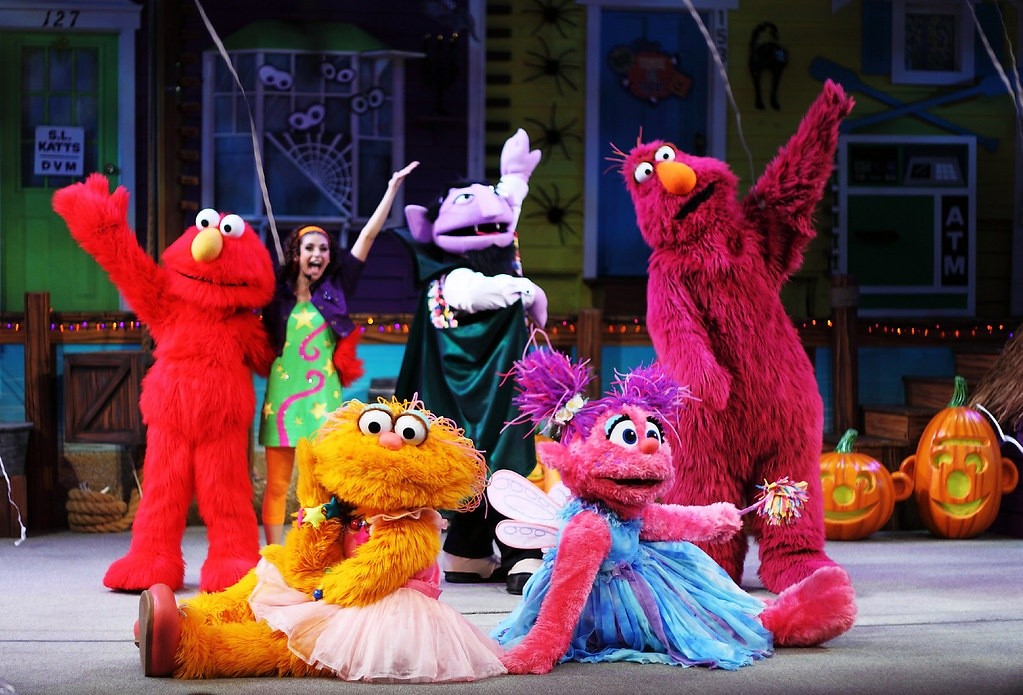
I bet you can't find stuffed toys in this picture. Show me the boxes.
[622,79,856,645]
[134,398,508,682]
[480,347,772,674]
[405,129,548,584]
[53,173,275,592]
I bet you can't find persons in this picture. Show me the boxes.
[258,161,420,544]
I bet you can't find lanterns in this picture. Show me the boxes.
[901,406,1019,538]
[820,451,912,541]
